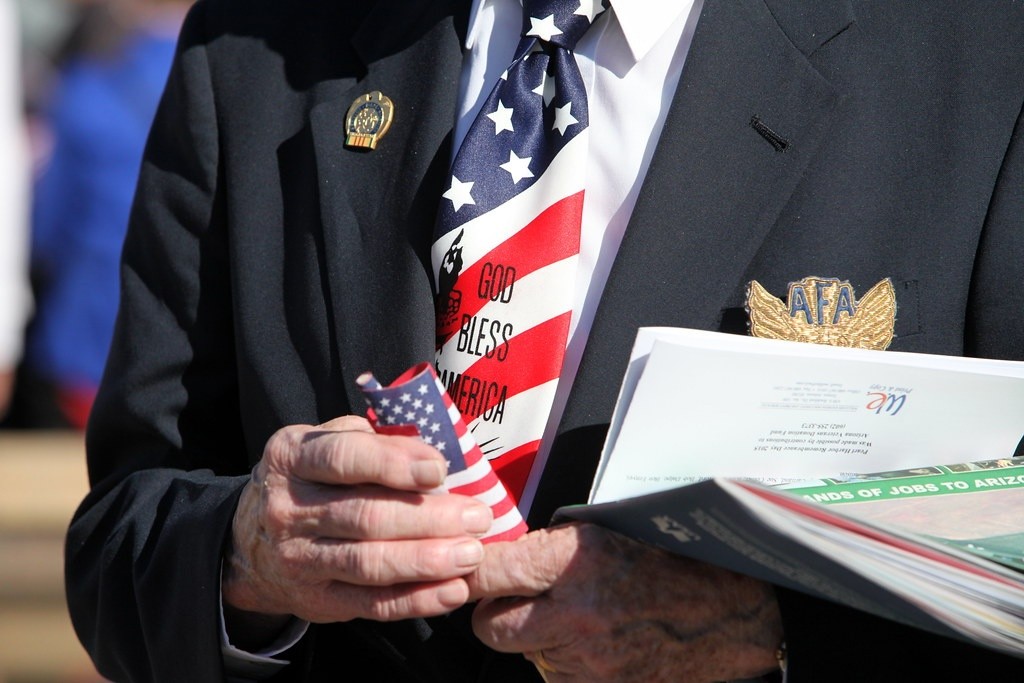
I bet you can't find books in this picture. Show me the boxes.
[551,324,1024,651]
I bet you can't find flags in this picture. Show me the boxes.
[354,361,528,546]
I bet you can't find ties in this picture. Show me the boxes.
[413,0,612,643]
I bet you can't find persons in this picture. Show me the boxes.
[0,0,191,425]
[64,0,1024,683]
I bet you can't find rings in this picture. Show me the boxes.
[535,650,555,671]
